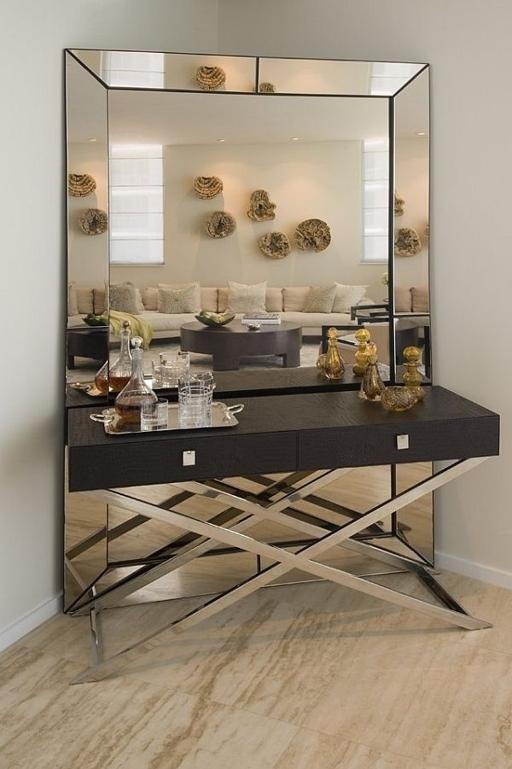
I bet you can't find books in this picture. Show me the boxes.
[241,313,282,325]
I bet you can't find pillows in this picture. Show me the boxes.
[395,286,430,313]
[109,278,373,315]
[68,278,107,316]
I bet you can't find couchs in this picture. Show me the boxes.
[108,277,378,349]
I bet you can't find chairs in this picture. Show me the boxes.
[395,313,429,381]
[318,304,390,366]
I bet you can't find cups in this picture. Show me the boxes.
[140,398,169,428]
[152,347,190,387]
[177,369,214,427]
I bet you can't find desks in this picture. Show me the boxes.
[66,381,504,665]
[65,366,431,564]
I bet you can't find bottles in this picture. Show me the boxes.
[115,336,159,425]
[357,347,426,411]
[109,320,133,393]
[316,326,378,379]
[94,359,109,394]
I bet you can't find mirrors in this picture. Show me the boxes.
[64,46,446,615]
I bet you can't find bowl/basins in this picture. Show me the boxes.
[194,314,236,328]
